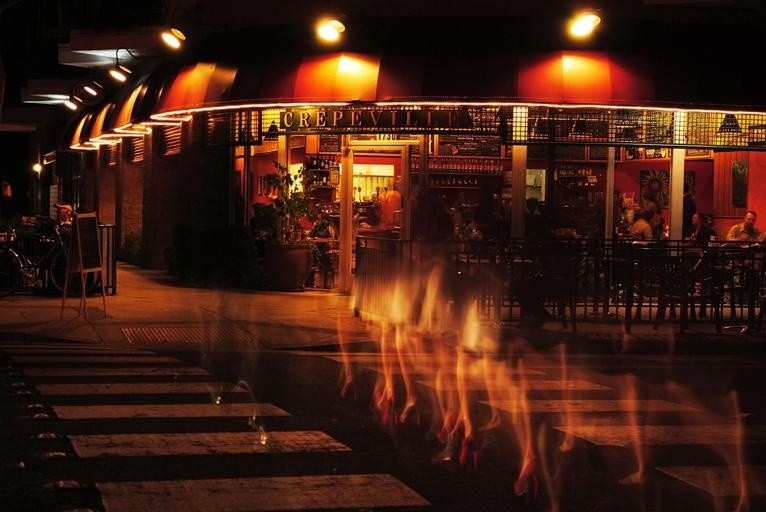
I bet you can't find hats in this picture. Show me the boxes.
[54,203,73,211]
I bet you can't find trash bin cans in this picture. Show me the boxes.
[356,231,412,321]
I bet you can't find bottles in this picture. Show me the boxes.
[310,155,337,170]
[411,158,489,173]
[358,201,364,216]
[308,172,329,187]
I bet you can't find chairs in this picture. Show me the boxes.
[353,217,765,334]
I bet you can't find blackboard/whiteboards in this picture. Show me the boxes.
[76,214,105,272]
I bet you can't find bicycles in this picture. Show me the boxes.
[0,219,102,298]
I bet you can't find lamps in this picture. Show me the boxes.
[108,45,159,82]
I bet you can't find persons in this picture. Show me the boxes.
[306,213,335,288]
[624,179,766,298]
[0,183,73,240]
[334,256,757,507]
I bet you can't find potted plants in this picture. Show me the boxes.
[259,153,322,292]
